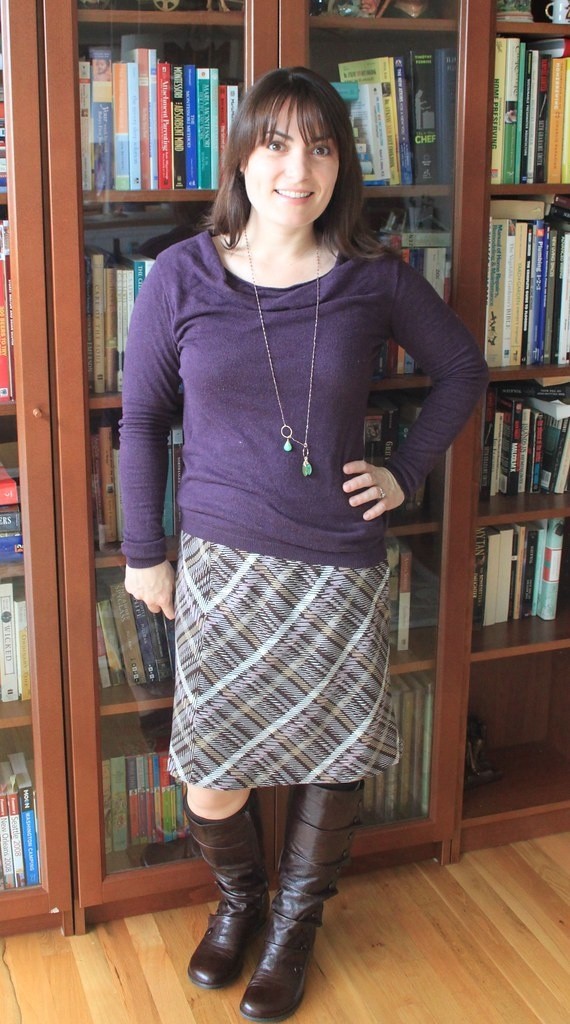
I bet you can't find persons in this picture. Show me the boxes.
[114,65,490,1022]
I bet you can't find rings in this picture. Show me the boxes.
[377,486,385,499]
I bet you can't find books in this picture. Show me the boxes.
[1,34,570,897]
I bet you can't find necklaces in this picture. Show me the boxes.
[242,224,322,478]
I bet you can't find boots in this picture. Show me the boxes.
[240,782,364,1022]
[140,707,189,865]
[189,793,274,988]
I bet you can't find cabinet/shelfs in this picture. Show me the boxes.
[0,0,569,937]
